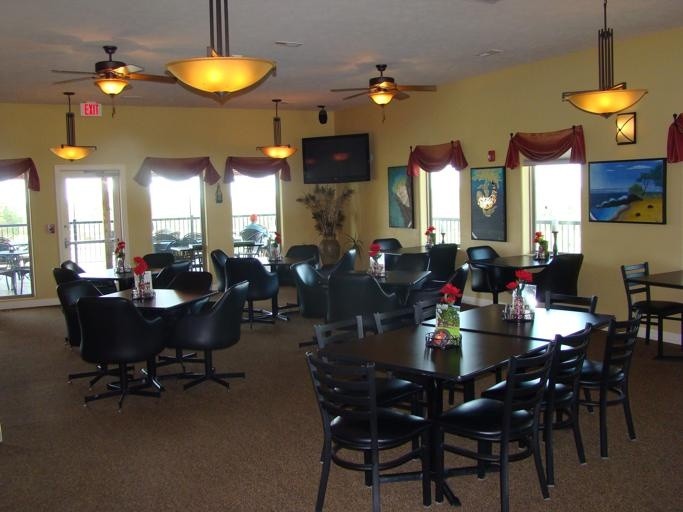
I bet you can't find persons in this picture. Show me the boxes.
[244,214,268,259]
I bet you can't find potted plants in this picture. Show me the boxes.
[293,184,353,265]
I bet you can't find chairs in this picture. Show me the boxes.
[164,280,251,392]
[621,260,682,359]
[486,322,592,489]
[306,350,433,511]
[51,252,215,391]
[210,235,467,328]
[1,213,279,297]
[544,290,598,316]
[577,309,642,461]
[435,341,556,512]
[75,297,166,412]
[314,295,444,350]
[306,227,598,465]
[468,243,585,306]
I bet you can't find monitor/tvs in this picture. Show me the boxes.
[302,132,375,184]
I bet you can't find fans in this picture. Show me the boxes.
[48,45,176,89]
[327,62,437,103]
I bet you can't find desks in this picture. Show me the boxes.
[318,323,553,505]
[420,302,617,453]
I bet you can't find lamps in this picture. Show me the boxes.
[160,1,276,100]
[93,77,127,96]
[215,184,223,204]
[47,90,97,161]
[255,98,297,158]
[559,1,649,118]
[367,88,395,105]
[616,110,637,145]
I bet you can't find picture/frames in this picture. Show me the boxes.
[386,165,415,228]
[586,157,667,224]
[470,166,507,243]
